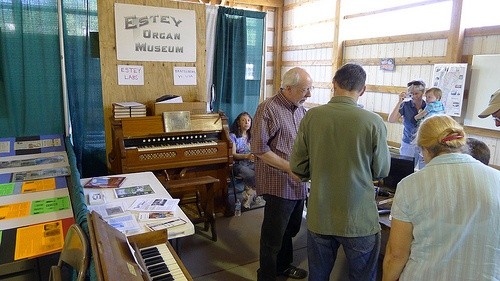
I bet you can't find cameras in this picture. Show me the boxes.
[406,94,413,98]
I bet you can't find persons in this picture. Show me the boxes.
[388,80,427,170]
[227,112,262,209]
[252,67,314,281]
[289,63,391,281]
[414,87,446,121]
[381,113,500,281]
[477,88,500,129]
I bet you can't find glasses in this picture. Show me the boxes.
[407,80,425,87]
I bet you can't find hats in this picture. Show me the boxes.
[478,89,500,118]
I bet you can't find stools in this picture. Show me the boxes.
[163,174,221,256]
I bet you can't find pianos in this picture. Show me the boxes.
[87,210,193,281]
[110,113,234,218]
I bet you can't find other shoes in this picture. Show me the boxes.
[277,265,307,279]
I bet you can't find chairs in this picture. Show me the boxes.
[49,224,91,281]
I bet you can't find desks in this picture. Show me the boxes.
[80,170,195,259]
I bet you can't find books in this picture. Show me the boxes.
[112,102,146,119]
[84,177,187,235]
[0,134,76,264]
[163,111,191,133]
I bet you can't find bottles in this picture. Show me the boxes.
[235,199,241,216]
